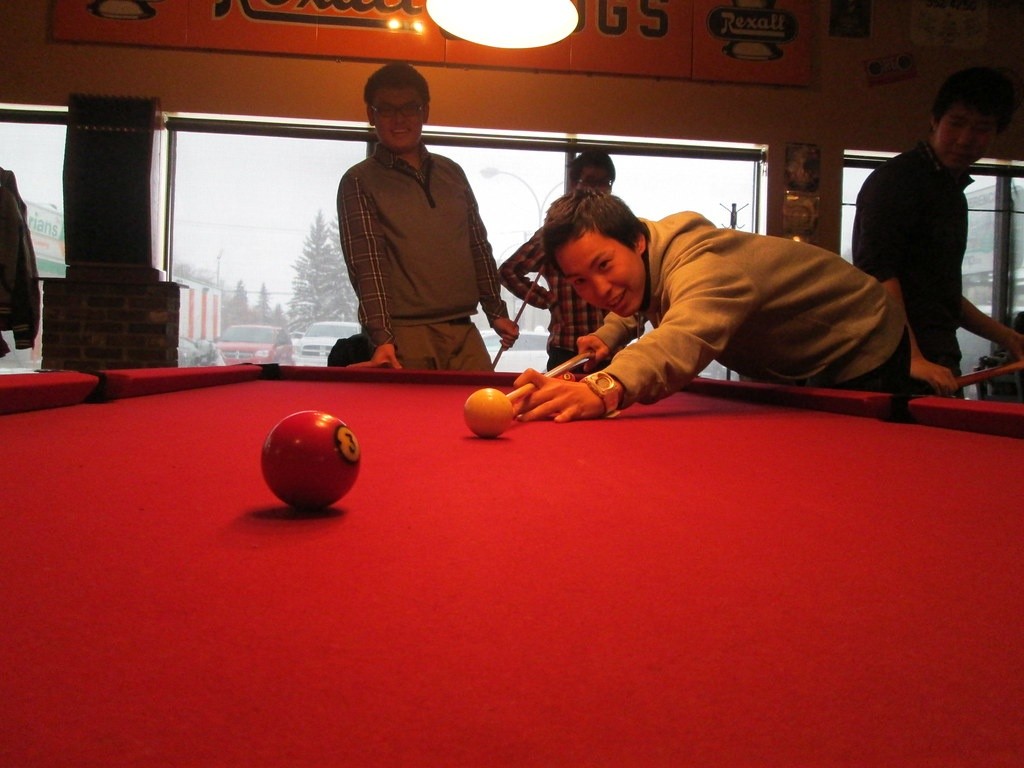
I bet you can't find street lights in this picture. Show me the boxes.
[481,168,563,227]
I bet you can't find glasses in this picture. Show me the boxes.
[577,177,612,187]
[370,100,424,117]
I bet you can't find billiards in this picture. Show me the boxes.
[464,388,513,438]
[261,411,361,509]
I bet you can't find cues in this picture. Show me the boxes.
[956,358,1024,389]
[492,263,548,374]
[505,352,594,407]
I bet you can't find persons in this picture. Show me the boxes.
[337,61,520,371]
[853,66,1024,394]
[512,187,912,421]
[497,150,626,373]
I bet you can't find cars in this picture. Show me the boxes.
[479,329,550,373]
[289,320,362,366]
[221,325,294,366]
[177,337,220,367]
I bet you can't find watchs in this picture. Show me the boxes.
[581,371,622,419]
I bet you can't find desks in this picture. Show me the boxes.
[0,363,1022,768]
